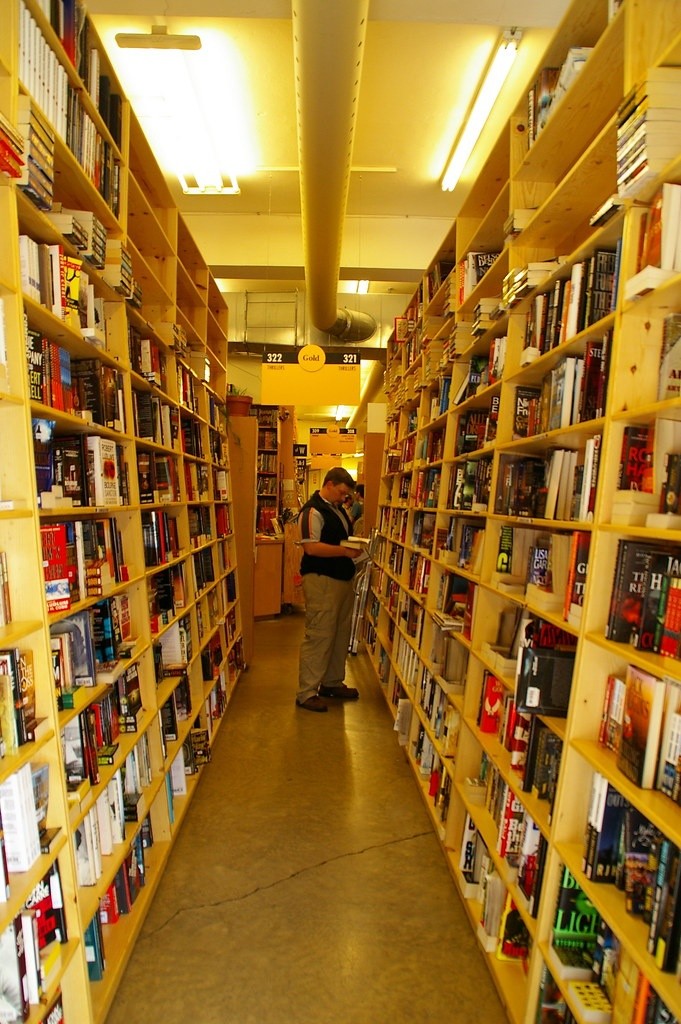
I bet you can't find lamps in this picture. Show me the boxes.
[439,29,522,193]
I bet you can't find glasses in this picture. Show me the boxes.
[354,492,356,494]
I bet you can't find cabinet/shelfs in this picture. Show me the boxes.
[0,0,681,1024]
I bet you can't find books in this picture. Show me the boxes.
[0,0,681,1024]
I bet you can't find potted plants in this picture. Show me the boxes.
[225,382,254,417]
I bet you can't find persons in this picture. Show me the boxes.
[349,485,366,523]
[293,467,359,711]
[342,494,353,523]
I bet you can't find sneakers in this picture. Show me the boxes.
[296,695,328,712]
[318,683,359,698]
